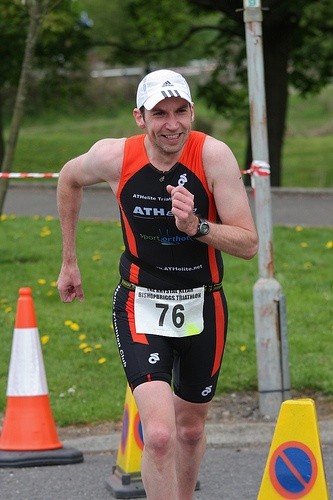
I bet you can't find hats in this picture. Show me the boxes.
[135,69,193,110]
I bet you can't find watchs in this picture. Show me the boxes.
[190,217,211,240]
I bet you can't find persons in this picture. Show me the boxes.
[56,69,259,500]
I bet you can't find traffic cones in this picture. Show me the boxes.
[256,397,328,499]
[105,378,202,500]
[0,287,85,469]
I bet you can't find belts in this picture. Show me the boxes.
[115,273,224,296]
